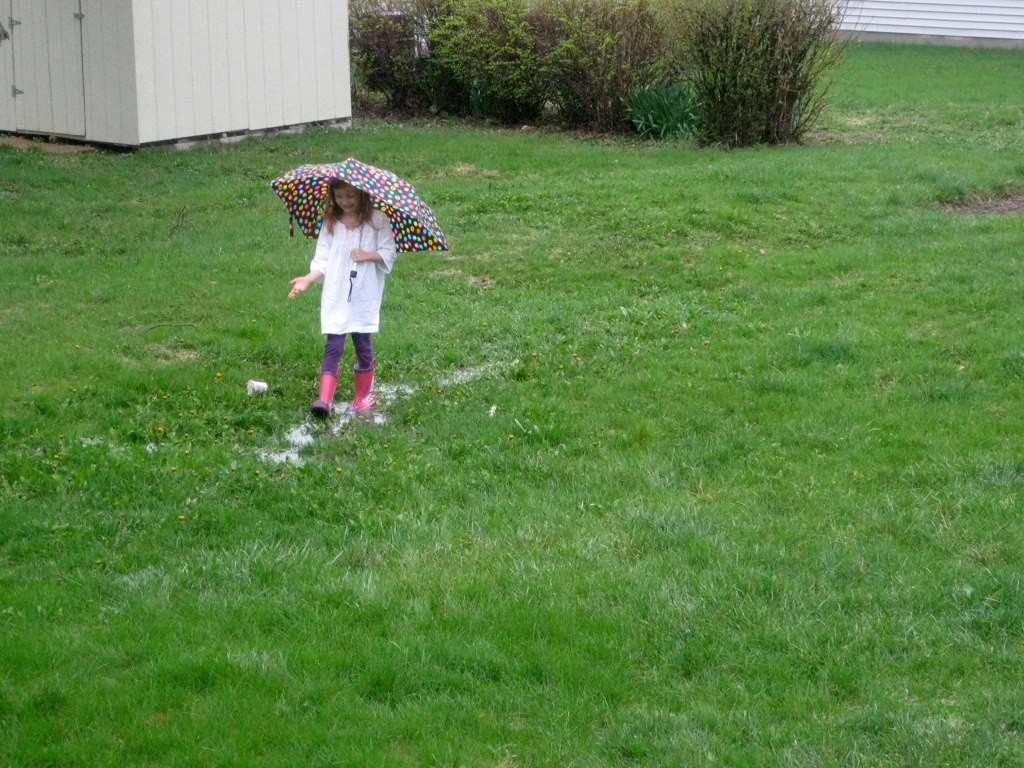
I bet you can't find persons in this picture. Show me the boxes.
[287,178,398,416]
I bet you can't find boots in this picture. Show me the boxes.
[349,358,376,411]
[310,369,337,419]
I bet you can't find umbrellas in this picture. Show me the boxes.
[270,157,449,300]
